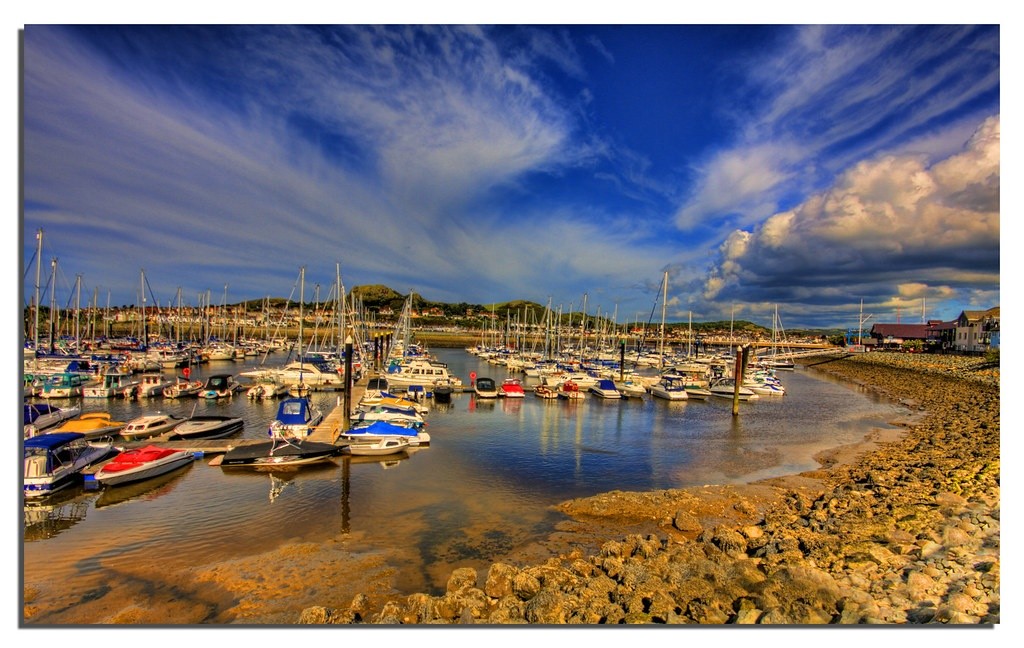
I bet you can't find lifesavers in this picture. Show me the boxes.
[470,372,477,379]
[205,392,218,398]
[183,367,191,376]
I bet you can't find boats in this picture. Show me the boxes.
[500,377,526,398]
[198,373,238,399]
[245,376,290,401]
[686,383,711,399]
[649,375,684,403]
[219,439,344,476]
[558,381,583,400]
[347,377,455,456]
[95,442,195,485]
[173,412,243,442]
[592,377,620,401]
[615,378,646,397]
[23,433,112,499]
[710,377,754,401]
[48,410,125,441]
[473,377,497,400]
[532,384,557,401]
[119,410,182,438]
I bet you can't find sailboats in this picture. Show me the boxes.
[465,266,795,382]
[23,225,459,434]
